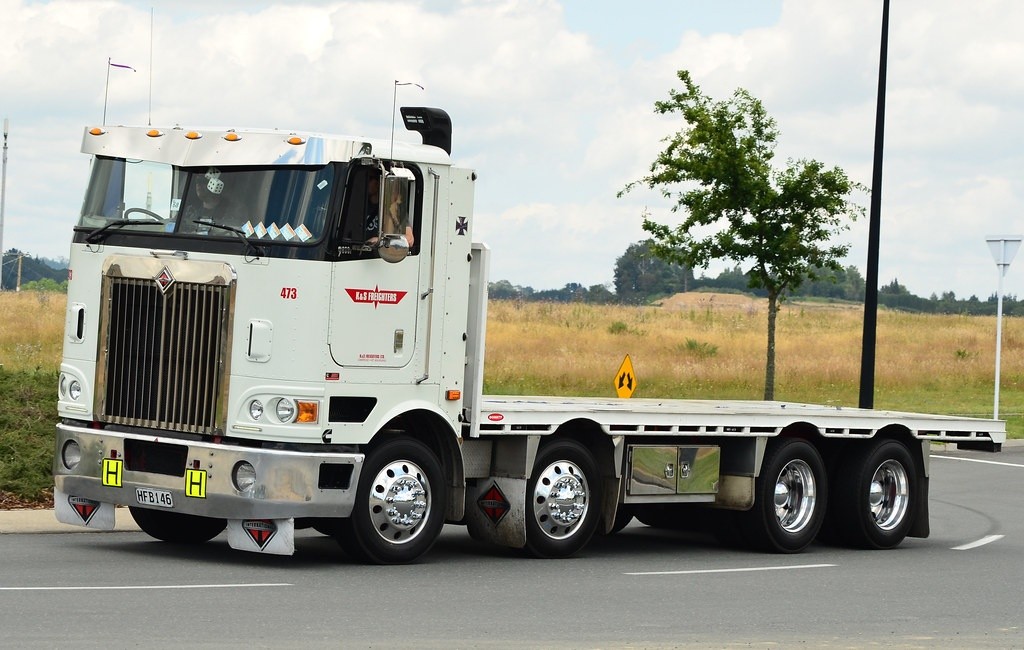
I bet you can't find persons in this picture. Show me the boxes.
[160,173,251,237]
[360,175,415,248]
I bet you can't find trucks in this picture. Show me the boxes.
[54,56,1008,564]
